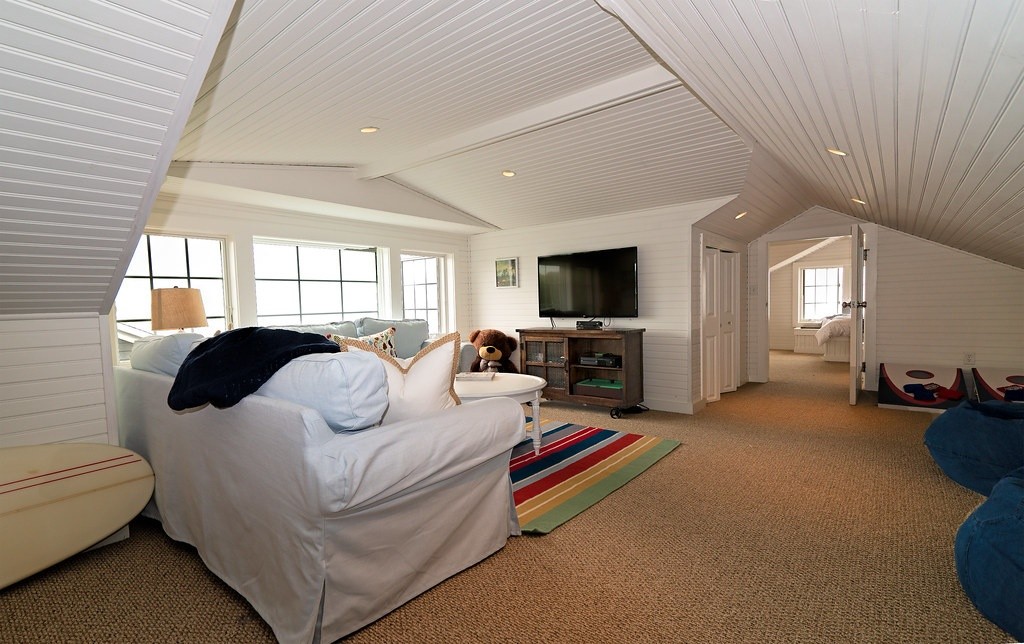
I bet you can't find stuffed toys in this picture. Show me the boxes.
[468,329,521,375]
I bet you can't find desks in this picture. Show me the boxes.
[453,372,547,457]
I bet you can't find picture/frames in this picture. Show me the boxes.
[494,258,519,287]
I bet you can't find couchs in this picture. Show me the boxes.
[113,365,526,644]
[339,331,461,425]
[419,339,478,373]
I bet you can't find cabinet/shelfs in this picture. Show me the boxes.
[516,327,645,411]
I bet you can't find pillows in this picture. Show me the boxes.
[265,321,358,340]
[253,350,391,433]
[357,317,429,359]
[325,325,398,358]
[130,331,206,378]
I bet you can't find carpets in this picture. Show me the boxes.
[508,416,681,535]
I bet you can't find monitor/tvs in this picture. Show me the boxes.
[538,246,638,318]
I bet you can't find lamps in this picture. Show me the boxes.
[151,286,209,333]
